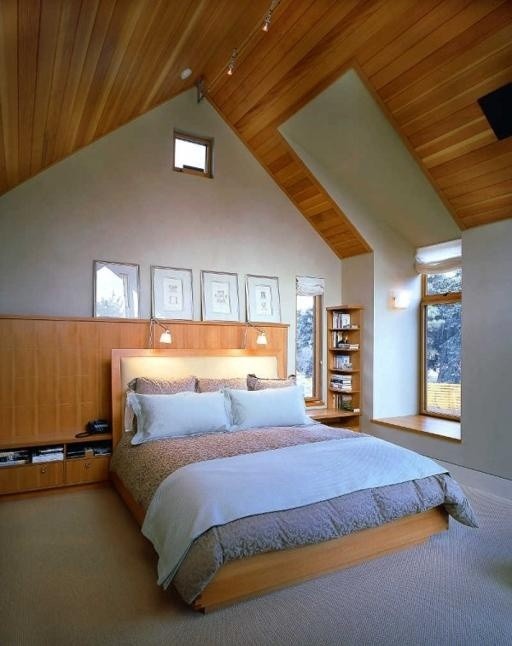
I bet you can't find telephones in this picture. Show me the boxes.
[87,421,108,434]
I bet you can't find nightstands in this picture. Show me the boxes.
[309,408,361,433]
[0,431,113,491]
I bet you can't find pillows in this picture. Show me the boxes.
[124,390,234,445]
[126,373,200,435]
[246,371,296,390]
[195,376,248,396]
[220,385,315,433]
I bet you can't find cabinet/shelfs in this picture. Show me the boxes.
[326,304,363,420]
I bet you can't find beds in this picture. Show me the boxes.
[109,347,481,615]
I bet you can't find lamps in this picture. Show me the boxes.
[240,322,267,349]
[390,289,411,308]
[147,317,172,348]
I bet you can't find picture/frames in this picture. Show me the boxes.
[200,269,241,321]
[90,259,140,318]
[244,273,281,323]
[150,264,195,320]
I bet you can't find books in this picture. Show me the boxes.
[328,311,359,409]
